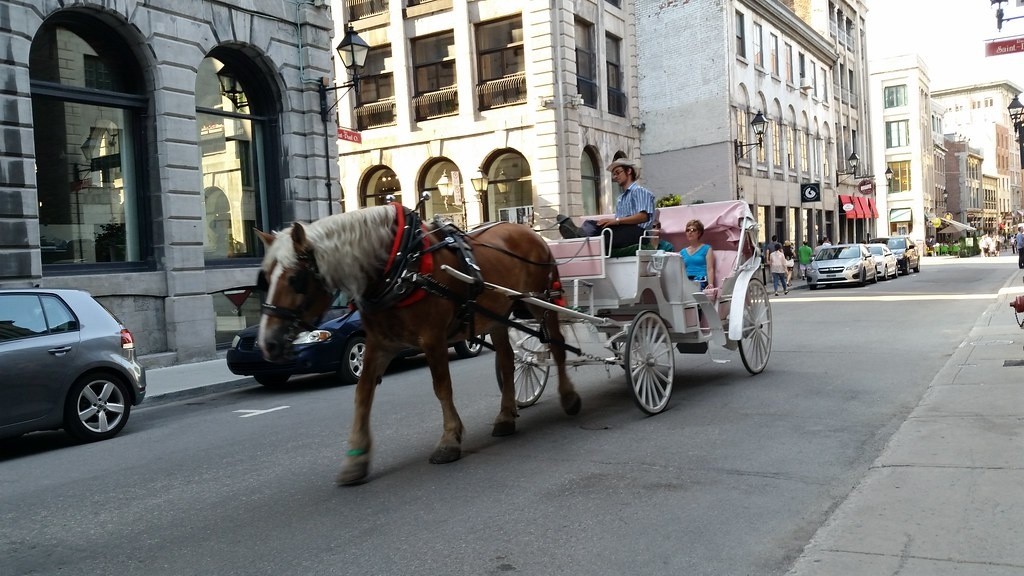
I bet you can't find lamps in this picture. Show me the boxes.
[936,189,949,208]
[735,109,769,163]
[885,166,893,187]
[836,151,860,187]
[319,23,371,122]
[571,94,584,108]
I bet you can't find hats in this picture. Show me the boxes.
[783,240,791,247]
[605,149,641,181]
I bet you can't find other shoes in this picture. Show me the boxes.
[775,292,778,296]
[787,282,790,287]
[784,291,788,295]
[557,214,586,239]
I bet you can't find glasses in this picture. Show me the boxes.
[652,223,661,228]
[686,228,699,232]
[612,169,625,176]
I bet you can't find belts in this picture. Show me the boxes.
[687,276,705,280]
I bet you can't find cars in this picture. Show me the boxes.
[225,289,486,389]
[0,286,148,444]
[867,242,898,281]
[806,243,878,290]
[867,235,921,275]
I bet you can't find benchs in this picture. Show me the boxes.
[587,228,666,300]
[679,250,746,326]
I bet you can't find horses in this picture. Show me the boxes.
[250,200,583,489]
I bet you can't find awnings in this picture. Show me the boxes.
[939,217,978,232]
[849,195,864,219]
[857,196,872,218]
[838,194,856,219]
[868,198,879,219]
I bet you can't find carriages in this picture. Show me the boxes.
[252,186,774,487]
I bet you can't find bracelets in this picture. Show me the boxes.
[615,217,620,224]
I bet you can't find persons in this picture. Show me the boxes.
[797,240,813,281]
[558,157,656,258]
[928,235,962,257]
[763,234,796,296]
[860,239,867,244]
[758,243,765,264]
[1007,226,1024,268]
[646,219,675,253]
[815,236,832,258]
[678,220,715,294]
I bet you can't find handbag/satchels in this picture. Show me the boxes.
[785,258,795,267]
